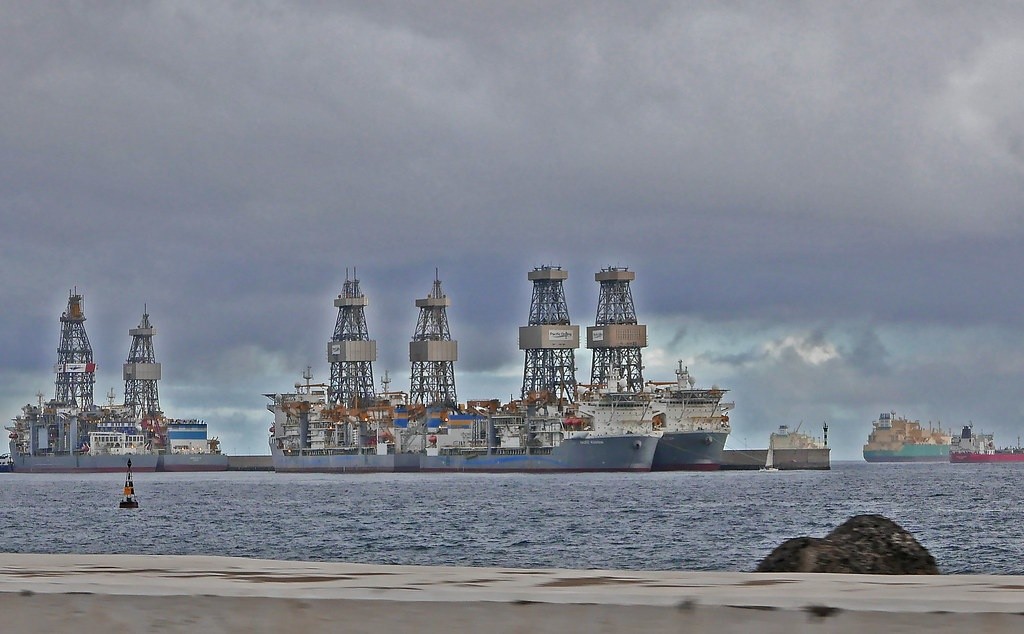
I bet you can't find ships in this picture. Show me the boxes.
[264,260,663,472]
[8,286,228,472]
[515,263,733,468]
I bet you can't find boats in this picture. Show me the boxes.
[861,413,949,460]
[722,423,831,469]
[949,423,1024,461]
